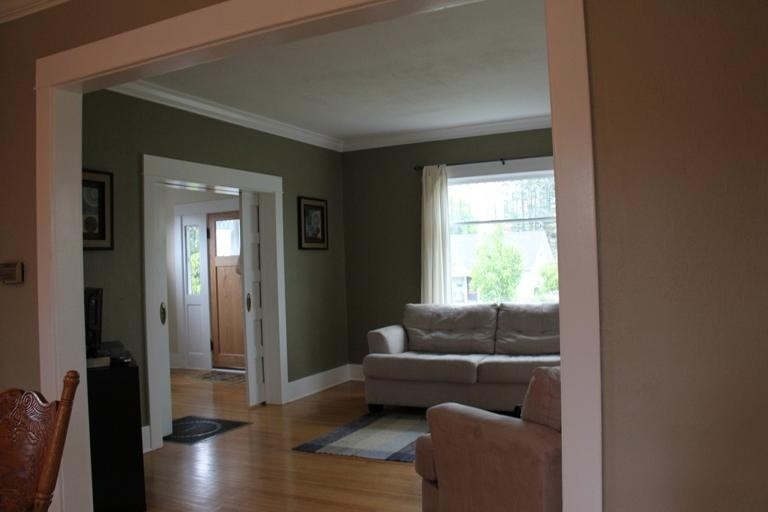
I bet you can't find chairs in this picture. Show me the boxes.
[0,370,79,511]
[407,364,564,510]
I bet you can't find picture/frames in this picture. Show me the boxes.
[81,168,114,251]
[295,194,329,249]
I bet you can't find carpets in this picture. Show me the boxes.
[165,415,252,446]
[192,369,247,386]
[291,406,431,465]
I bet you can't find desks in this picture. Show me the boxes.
[86,340,146,510]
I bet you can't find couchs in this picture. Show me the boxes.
[358,300,560,413]
